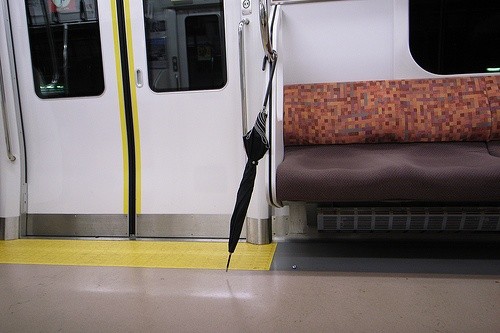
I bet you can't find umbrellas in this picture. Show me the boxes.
[226,50,278,272]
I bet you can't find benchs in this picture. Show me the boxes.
[482,74,500,159]
[276,75,500,241]
